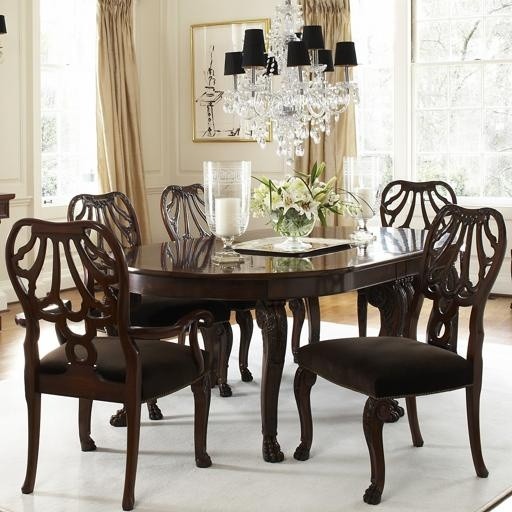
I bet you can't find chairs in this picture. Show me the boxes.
[161,182,307,381]
[66,191,238,421]
[6,217,221,510]
[291,205,506,506]
[358,179,458,341]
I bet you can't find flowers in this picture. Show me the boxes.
[250,160,365,237]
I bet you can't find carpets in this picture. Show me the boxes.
[0,316,512,512]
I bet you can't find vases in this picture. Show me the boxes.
[267,211,317,251]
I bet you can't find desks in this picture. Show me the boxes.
[102,225,463,465]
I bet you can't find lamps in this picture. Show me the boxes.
[225,0,360,172]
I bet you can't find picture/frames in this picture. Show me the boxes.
[189,18,272,144]
